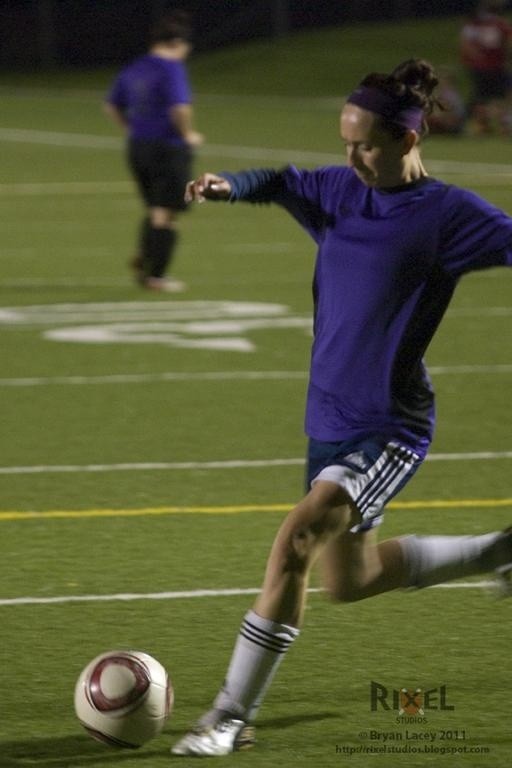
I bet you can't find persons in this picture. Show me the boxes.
[106,10,206,294]
[424,66,470,134]
[171,59,511,756]
[459,4,512,135]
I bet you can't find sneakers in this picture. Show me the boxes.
[170,707,248,758]
[129,255,189,294]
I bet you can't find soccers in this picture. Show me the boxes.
[75,650,175,750]
